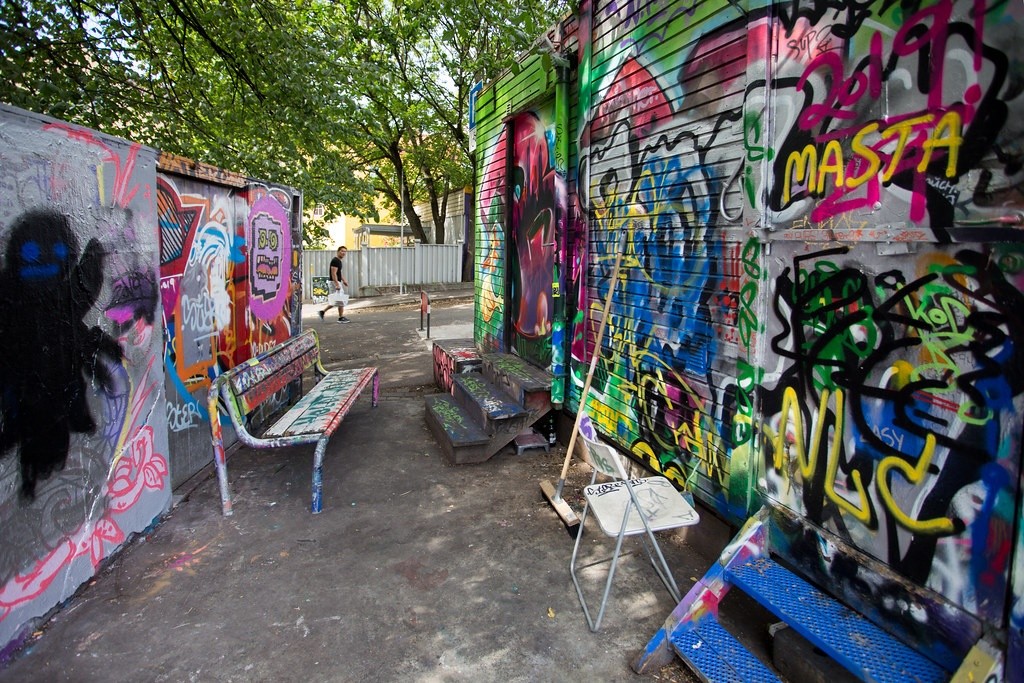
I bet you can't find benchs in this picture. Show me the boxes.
[207,328,380,518]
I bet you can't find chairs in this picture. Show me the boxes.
[570,411,701,636]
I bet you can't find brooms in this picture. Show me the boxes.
[538,228,628,538]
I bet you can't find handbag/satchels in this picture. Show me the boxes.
[327,289,349,307]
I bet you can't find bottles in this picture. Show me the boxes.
[547,415,556,446]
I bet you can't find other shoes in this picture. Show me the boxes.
[337,317,351,324]
[317,310,325,320]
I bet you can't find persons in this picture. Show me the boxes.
[318,246,350,324]
